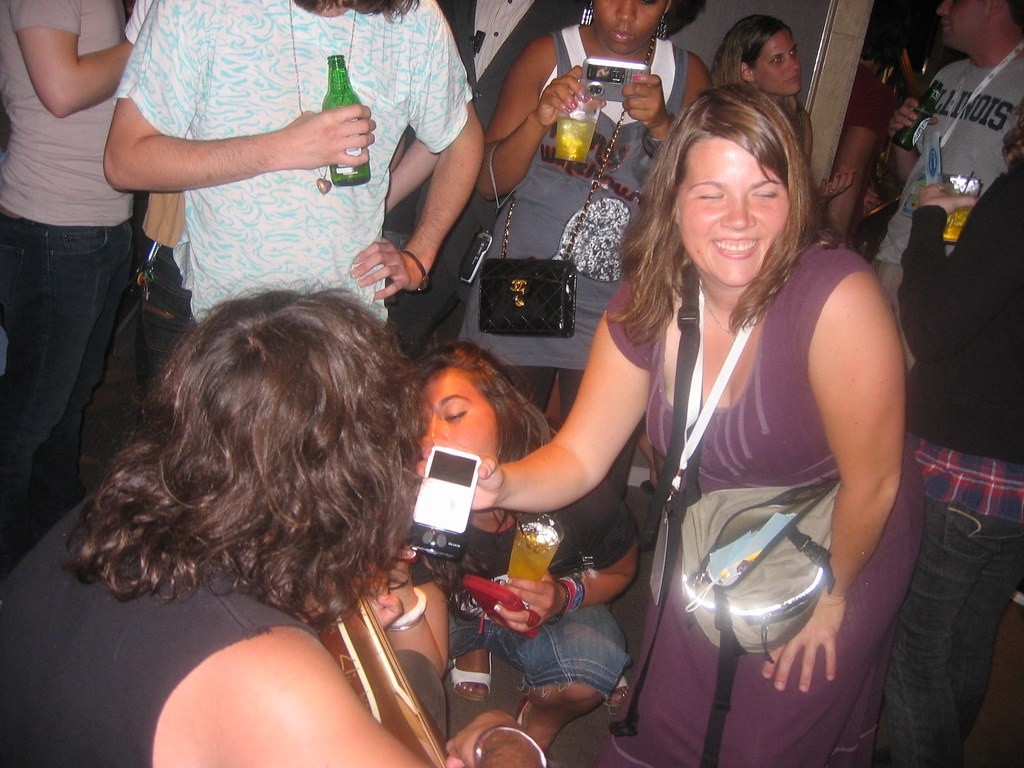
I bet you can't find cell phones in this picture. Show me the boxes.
[414,447,482,535]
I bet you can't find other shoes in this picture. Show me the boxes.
[512,697,530,727]
[32,494,78,530]
[0,535,39,578]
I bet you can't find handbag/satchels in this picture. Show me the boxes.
[680,477,842,652]
[479,258,578,338]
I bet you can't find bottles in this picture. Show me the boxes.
[321,55,371,186]
[892,79,944,151]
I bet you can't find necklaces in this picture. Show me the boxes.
[289,1,359,195]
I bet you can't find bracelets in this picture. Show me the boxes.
[382,582,428,632]
[642,114,679,156]
[474,722,547,768]
[403,250,429,291]
[551,575,585,624]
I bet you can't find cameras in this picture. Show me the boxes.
[578,56,649,103]
[459,229,492,285]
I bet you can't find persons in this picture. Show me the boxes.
[0,0,857,561]
[388,341,640,751]
[413,83,923,768]
[825,0,1024,768]
[0,284,548,768]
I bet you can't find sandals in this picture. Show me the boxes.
[601,675,629,706]
[448,652,492,701]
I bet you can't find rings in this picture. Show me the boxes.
[527,609,540,626]
[829,190,833,193]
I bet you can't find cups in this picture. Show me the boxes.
[937,173,981,244]
[555,94,603,163]
[507,513,565,583]
